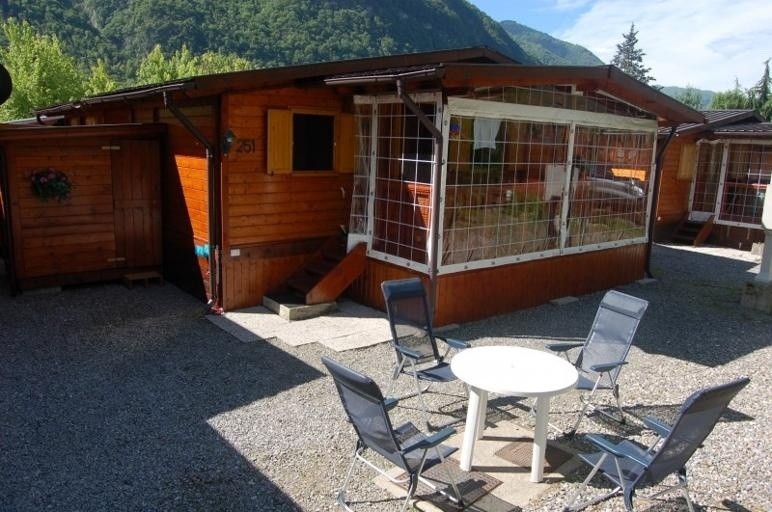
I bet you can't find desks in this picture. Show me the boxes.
[450,344,578,484]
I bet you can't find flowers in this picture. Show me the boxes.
[28,167,72,202]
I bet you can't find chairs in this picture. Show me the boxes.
[561,375,752,512]
[380,277,475,433]
[320,353,464,512]
[528,288,650,440]
